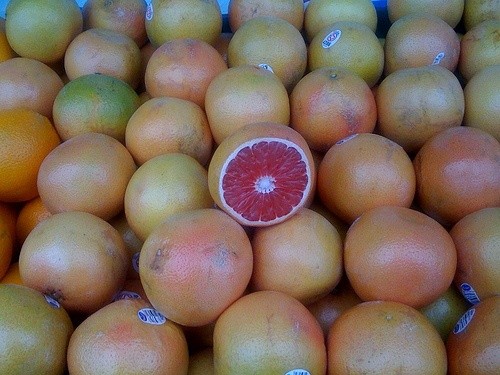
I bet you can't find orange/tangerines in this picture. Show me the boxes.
[0,0,500,375]
